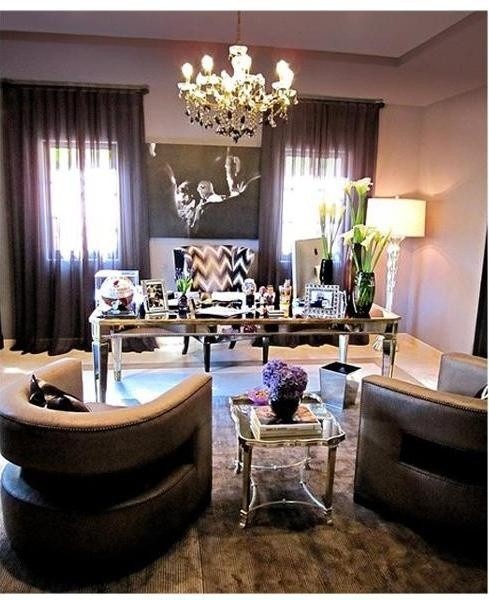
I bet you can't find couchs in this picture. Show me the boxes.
[0,357,213,585]
[353,352,487,543]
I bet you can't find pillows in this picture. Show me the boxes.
[23,371,90,414]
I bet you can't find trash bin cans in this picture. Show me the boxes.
[320,361,362,410]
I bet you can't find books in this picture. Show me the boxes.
[197,305,250,317]
[248,404,322,439]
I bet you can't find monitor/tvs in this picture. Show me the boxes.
[292,237,328,298]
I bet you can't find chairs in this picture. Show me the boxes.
[173,244,269,372]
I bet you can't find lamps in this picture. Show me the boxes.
[175,11,299,143]
[364,197,427,351]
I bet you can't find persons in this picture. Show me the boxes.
[311,293,324,307]
[321,299,330,308]
[256,303,267,314]
[145,143,261,240]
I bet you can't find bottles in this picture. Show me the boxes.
[279,278,292,303]
[279,303,290,317]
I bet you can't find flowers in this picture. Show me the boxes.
[342,178,374,226]
[319,200,346,258]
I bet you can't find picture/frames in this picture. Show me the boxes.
[303,284,339,318]
[141,278,169,312]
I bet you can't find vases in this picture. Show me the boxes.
[352,271,377,320]
[340,223,391,271]
[320,258,334,286]
[343,244,357,293]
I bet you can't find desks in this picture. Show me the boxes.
[89,296,400,402]
[230,392,346,527]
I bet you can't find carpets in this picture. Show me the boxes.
[82,354,432,455]
